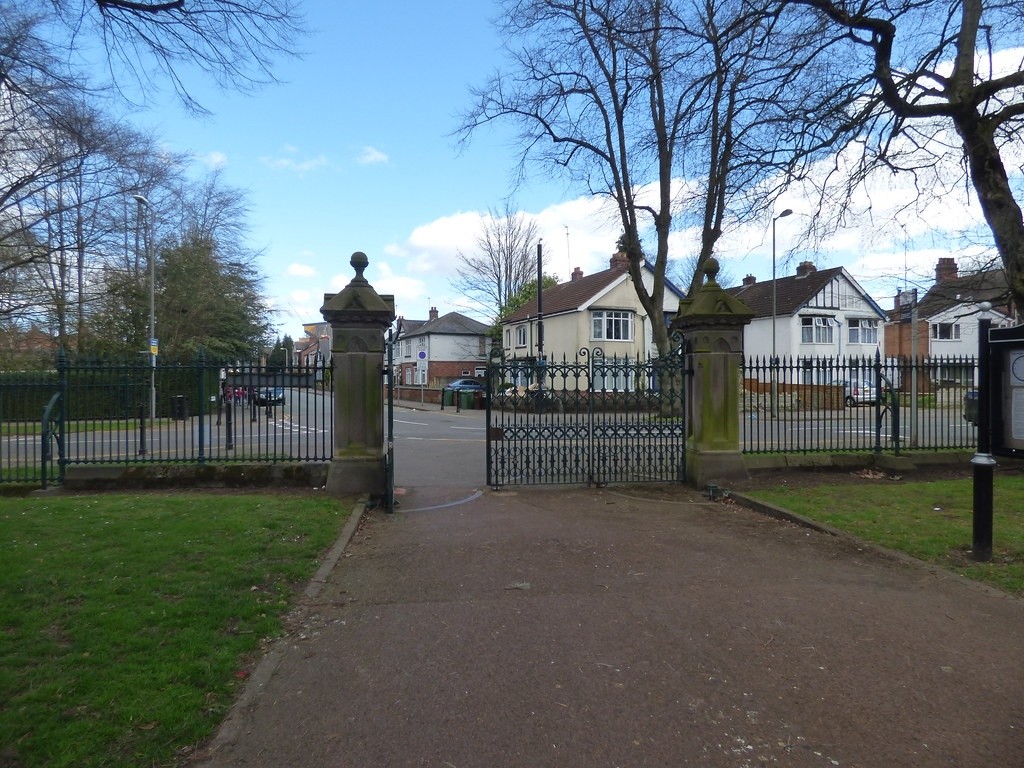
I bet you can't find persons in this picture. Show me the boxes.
[226,387,254,407]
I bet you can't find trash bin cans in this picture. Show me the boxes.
[171,395,190,421]
[460,389,476,410]
[443,388,455,406]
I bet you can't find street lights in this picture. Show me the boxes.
[771,207,794,419]
[132,193,158,419]
[280,347,289,373]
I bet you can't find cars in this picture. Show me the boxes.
[254,386,286,406]
[961,390,979,428]
[826,378,877,408]
[444,378,486,393]
